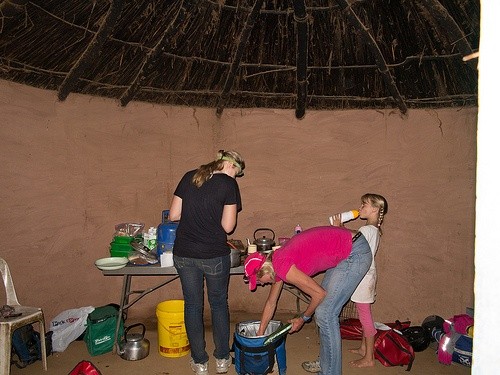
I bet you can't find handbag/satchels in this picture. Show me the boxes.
[384,314,474,368]
[32,330,53,359]
[374,329,414,371]
[48,306,95,352]
[230,320,287,375]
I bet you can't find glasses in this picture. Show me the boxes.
[235,173,244,177]
[243,275,249,284]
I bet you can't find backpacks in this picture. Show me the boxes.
[9,324,39,369]
[83,305,126,356]
[340,318,379,340]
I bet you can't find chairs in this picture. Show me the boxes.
[0,258,47,375]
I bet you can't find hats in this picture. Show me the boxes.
[243,251,267,290]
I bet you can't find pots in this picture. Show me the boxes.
[230,248,241,267]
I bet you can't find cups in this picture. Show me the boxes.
[248,245,256,255]
[272,246,282,251]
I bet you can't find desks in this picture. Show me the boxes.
[104,262,320,354]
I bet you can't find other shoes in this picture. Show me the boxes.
[302,360,321,373]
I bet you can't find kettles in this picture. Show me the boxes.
[246,228,277,253]
[115,323,150,361]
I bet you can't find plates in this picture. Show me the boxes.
[128,255,159,265]
[94,256,129,270]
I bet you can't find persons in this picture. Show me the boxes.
[332,193,388,368]
[245,225,372,375]
[168,149,244,375]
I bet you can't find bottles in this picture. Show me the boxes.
[294,224,302,234]
[330,210,360,226]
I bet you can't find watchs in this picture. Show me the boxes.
[301,313,311,321]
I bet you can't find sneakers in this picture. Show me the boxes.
[215,354,232,374]
[189,358,209,375]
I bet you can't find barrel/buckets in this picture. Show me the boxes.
[155,299,191,357]
